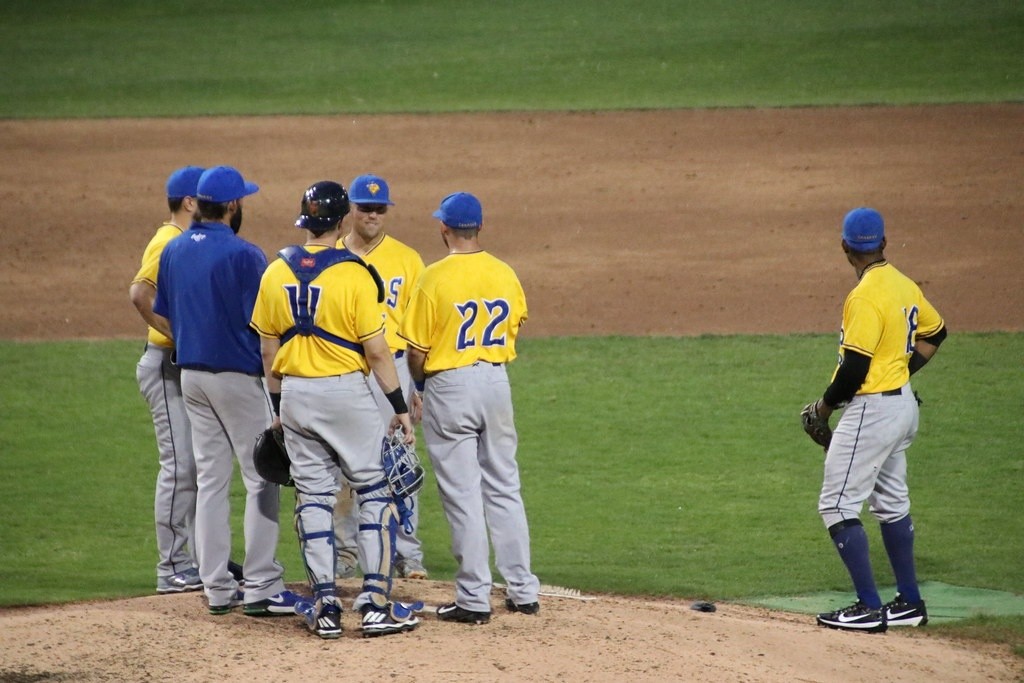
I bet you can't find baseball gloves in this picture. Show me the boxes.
[252,425,296,488]
[170,349,180,373]
[801,400,833,446]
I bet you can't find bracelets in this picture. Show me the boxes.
[384,386,408,415]
[414,378,425,391]
[269,392,281,416]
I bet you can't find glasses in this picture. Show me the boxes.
[355,203,387,214]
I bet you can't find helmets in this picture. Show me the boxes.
[294,180,350,231]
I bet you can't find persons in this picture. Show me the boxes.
[335,172,428,580]
[815,206,947,633]
[250,182,421,640]
[151,166,315,616]
[129,165,234,594]
[395,193,541,624]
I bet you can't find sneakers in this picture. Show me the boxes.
[362,604,423,638]
[885,592,928,627]
[816,599,887,632]
[156,567,204,593]
[227,560,246,584]
[243,590,317,615]
[208,589,245,615]
[305,605,342,639]
[506,597,538,615]
[436,602,490,625]
[404,570,429,579]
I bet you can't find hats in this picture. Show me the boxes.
[348,174,396,206]
[166,165,207,198]
[196,166,260,202]
[433,192,482,228]
[843,207,884,252]
